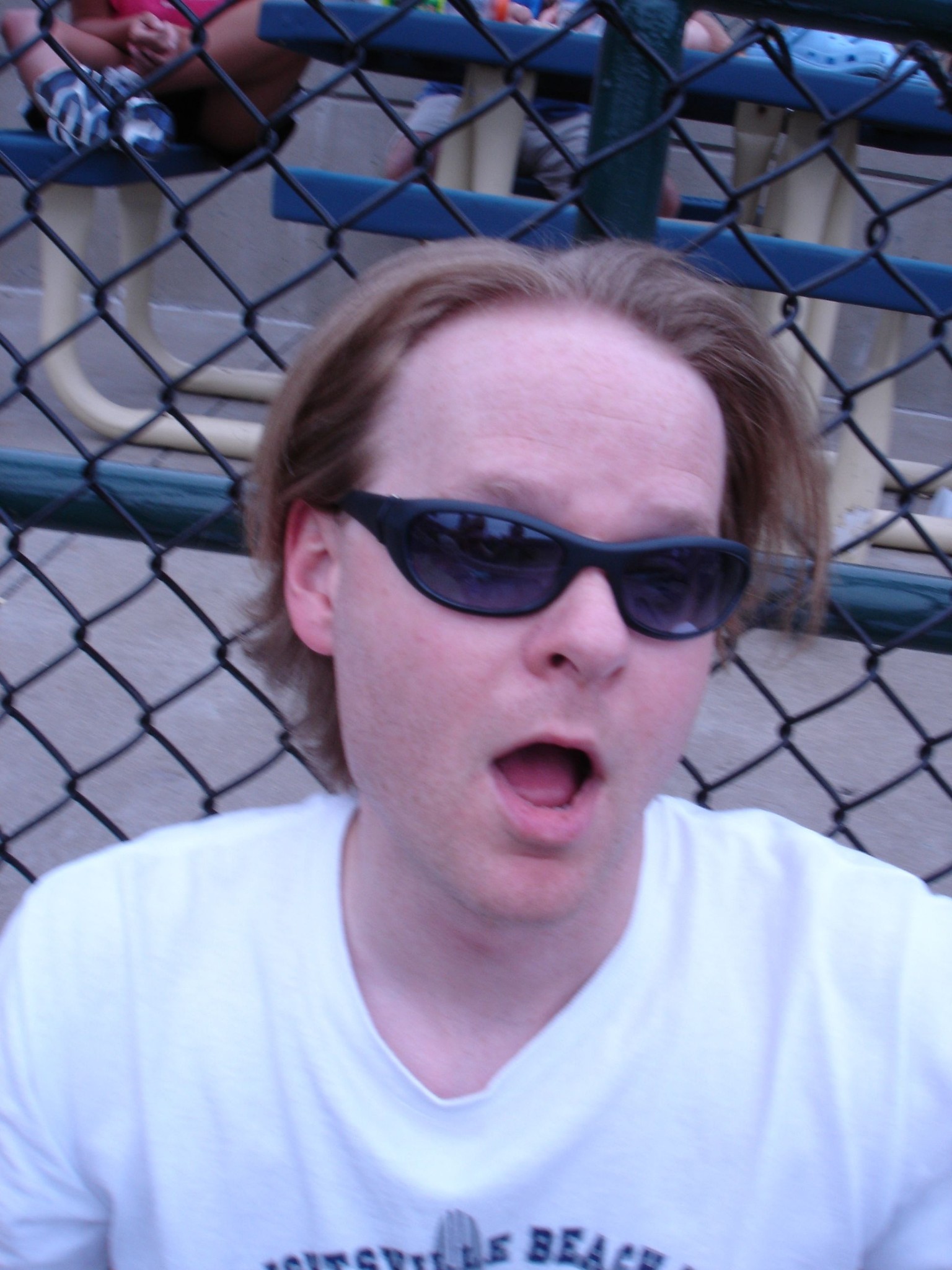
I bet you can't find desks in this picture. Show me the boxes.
[257,2,952,436]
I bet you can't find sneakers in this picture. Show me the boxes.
[24,65,175,162]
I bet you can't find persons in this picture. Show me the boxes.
[0,0,309,160]
[0,239,952,1269]
[385,0,733,219]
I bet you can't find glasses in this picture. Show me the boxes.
[324,487,753,640]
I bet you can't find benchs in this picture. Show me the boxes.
[1,117,952,564]
